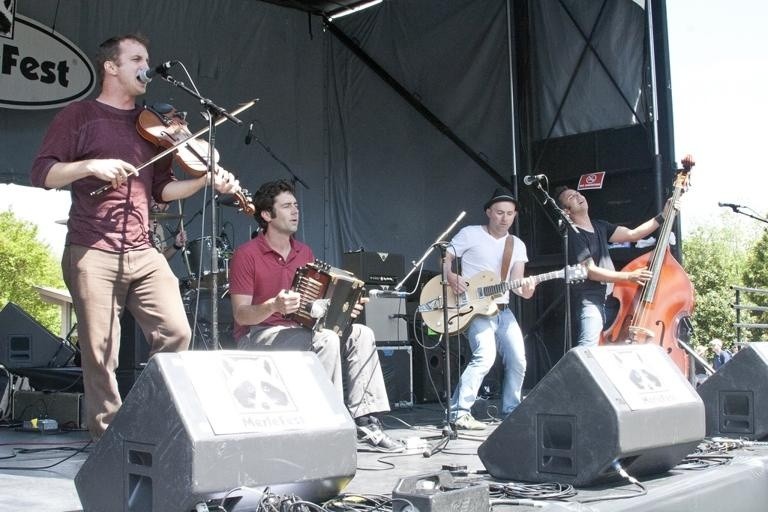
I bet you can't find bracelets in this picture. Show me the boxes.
[654,213,665,225]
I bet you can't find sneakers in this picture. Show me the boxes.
[356,415,404,453]
[444,413,484,430]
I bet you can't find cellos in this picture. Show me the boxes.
[599,156,695,380]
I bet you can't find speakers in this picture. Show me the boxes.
[77,350,359,512]
[0,300,75,368]
[477,345,706,488]
[409,321,446,402]
[696,343,767,442]
[351,284,410,345]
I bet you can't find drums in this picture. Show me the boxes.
[185,235,232,287]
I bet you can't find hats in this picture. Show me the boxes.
[484,187,517,209]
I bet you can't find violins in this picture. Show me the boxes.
[137,102,257,217]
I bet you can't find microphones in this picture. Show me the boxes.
[718,200,741,209]
[524,174,543,185]
[139,59,176,81]
[370,289,409,299]
[244,122,254,145]
[389,315,411,318]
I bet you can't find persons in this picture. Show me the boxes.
[709,339,729,371]
[554,186,681,349]
[230,179,402,450]
[443,188,539,431]
[29,33,236,444]
[730,343,739,358]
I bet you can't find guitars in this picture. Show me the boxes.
[418,263,588,334]
